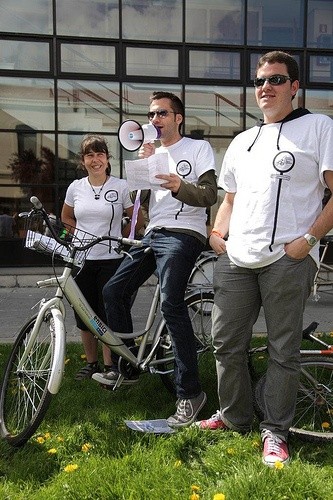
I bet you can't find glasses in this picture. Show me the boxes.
[253,74,293,86]
[147,111,175,120]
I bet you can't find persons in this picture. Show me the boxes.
[62,136,144,381]
[0,207,18,238]
[93,92,217,429]
[190,51,333,465]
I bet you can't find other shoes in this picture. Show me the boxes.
[103,366,116,389]
[74,364,100,380]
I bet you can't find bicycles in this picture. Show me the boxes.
[253,321,333,443]
[1,195,213,449]
[187,251,220,316]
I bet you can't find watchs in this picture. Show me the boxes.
[304,234,317,246]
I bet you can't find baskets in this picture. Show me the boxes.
[23,212,97,268]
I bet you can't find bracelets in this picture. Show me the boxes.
[212,230,222,239]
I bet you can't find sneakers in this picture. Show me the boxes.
[166,390,207,427]
[190,409,230,433]
[91,368,139,386]
[260,428,290,468]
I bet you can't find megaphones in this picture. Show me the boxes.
[118,119,161,152]
